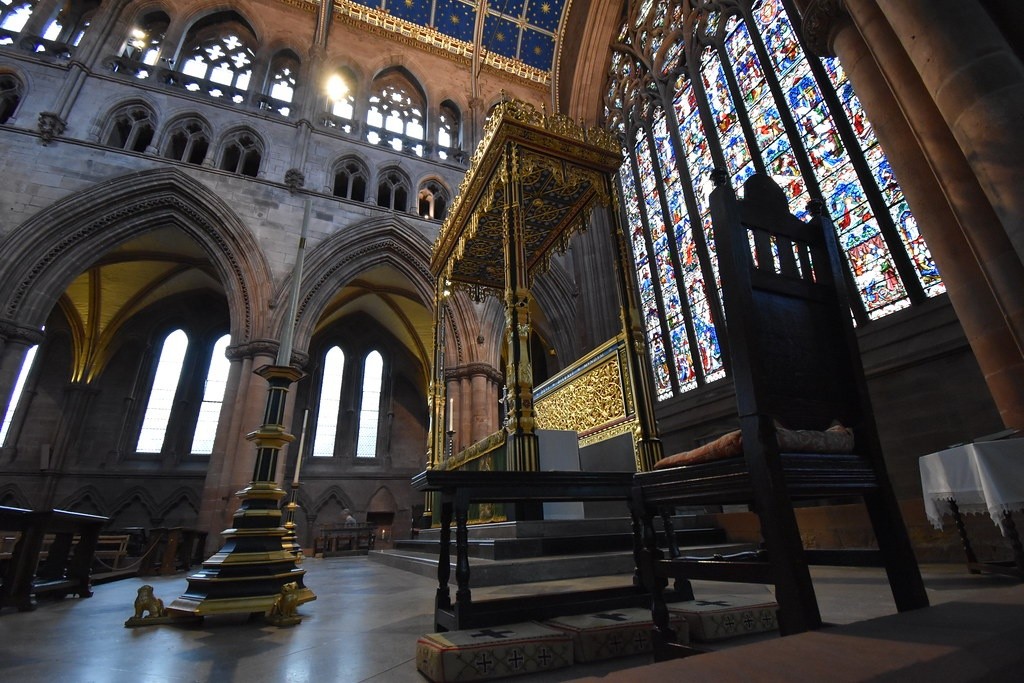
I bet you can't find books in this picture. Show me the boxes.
[973,428,1014,442]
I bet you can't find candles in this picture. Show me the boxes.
[283,502,297,511]
[284,521,294,528]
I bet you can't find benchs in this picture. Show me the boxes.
[12,531,130,571]
[320,536,376,552]
[100,527,150,554]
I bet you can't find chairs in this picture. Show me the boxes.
[629,174,932,665]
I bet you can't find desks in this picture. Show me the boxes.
[319,526,379,556]
[0,504,116,608]
[918,437,1024,577]
[322,522,376,550]
[412,471,696,635]
[134,527,208,577]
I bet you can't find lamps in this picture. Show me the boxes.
[546,347,557,356]
